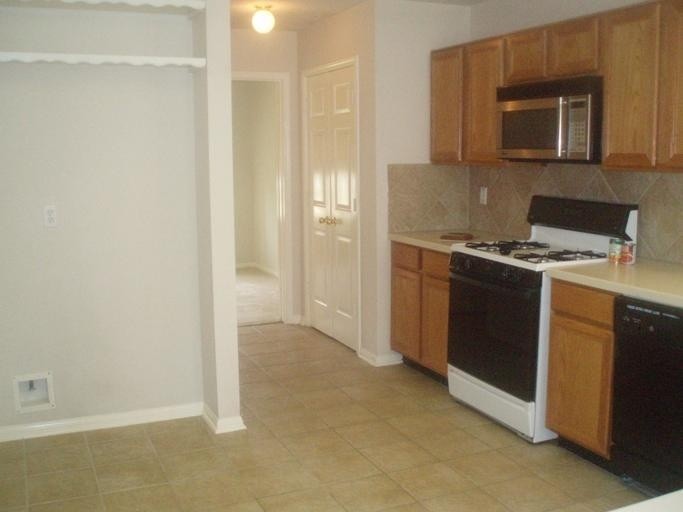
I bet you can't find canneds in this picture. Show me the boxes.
[622,242,636,264]
[609,238,623,264]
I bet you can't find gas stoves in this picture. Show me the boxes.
[448,238,608,289]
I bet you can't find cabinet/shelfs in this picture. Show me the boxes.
[544,278,621,461]
[389,241,449,379]
[504,13,601,87]
[602,0,682,174]
[430,35,504,168]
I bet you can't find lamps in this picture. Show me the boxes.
[251,6,275,34]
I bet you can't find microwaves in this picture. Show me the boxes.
[495,93,596,164]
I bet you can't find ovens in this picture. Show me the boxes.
[445,275,540,405]
